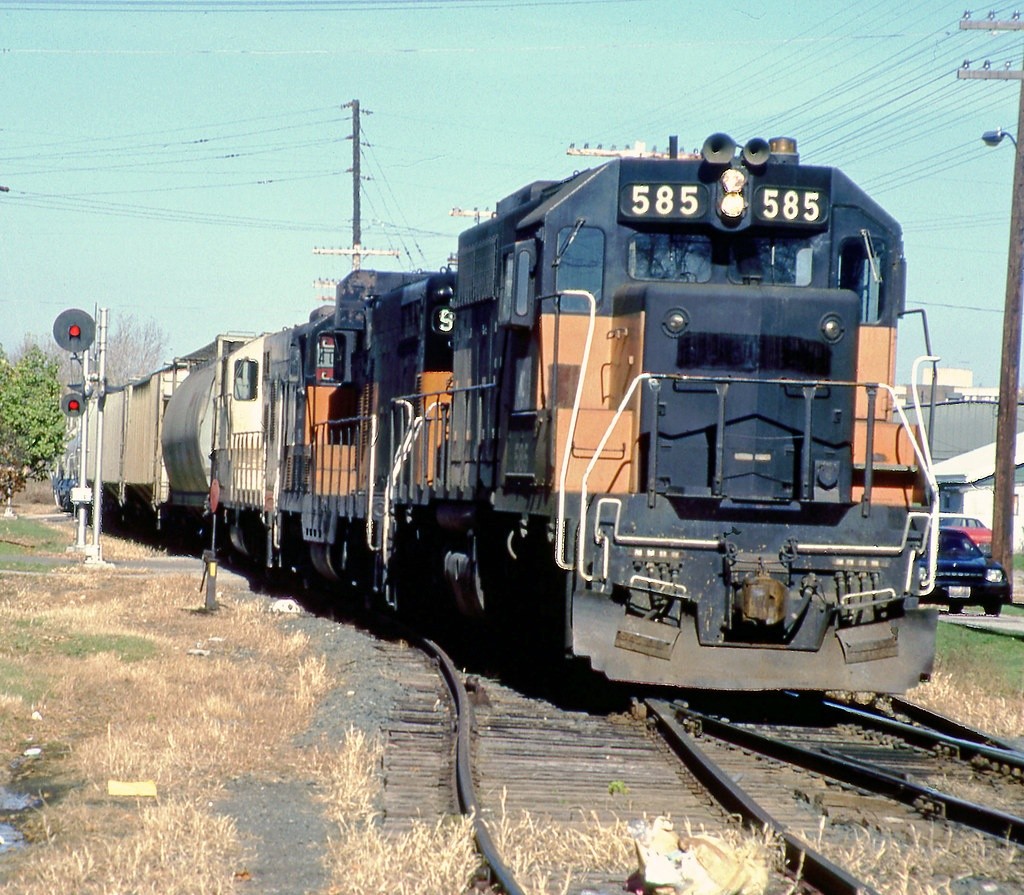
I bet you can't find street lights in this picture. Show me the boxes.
[981,131,1024,604]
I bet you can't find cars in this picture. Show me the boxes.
[939,517,992,557]
[917,528,1011,614]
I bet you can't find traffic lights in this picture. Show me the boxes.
[62,393,86,416]
[53,308,96,353]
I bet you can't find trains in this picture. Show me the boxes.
[52,133,938,691]
